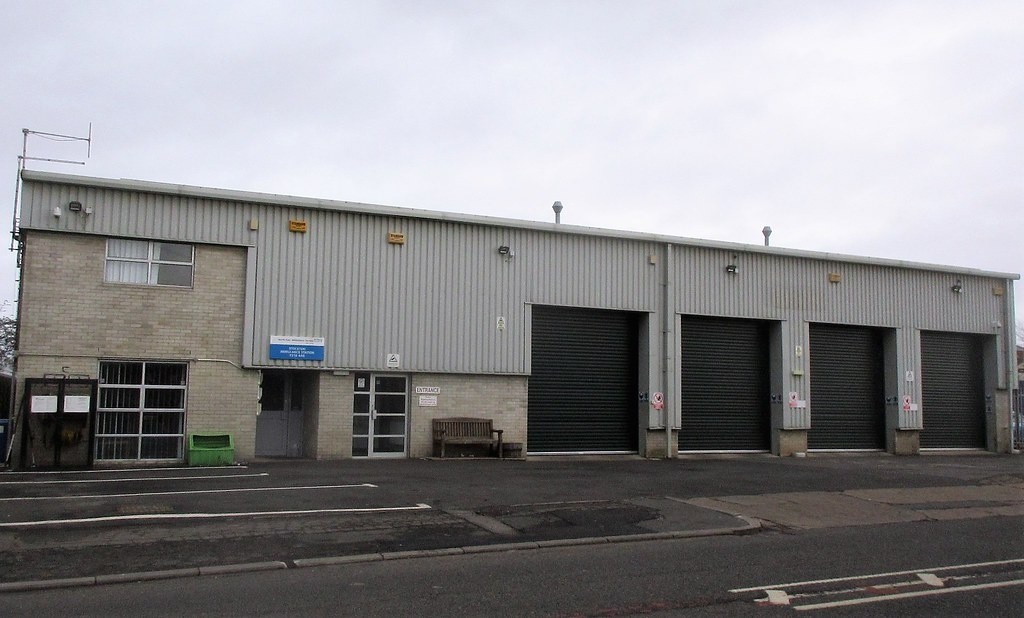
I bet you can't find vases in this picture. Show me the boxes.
[503,443,523,458]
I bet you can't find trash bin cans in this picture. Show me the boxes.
[186,431,234,467]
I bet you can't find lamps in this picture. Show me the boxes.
[69,201,81,211]
[725,265,737,272]
[499,245,509,254]
[953,285,962,293]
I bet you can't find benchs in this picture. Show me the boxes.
[432,416,503,458]
